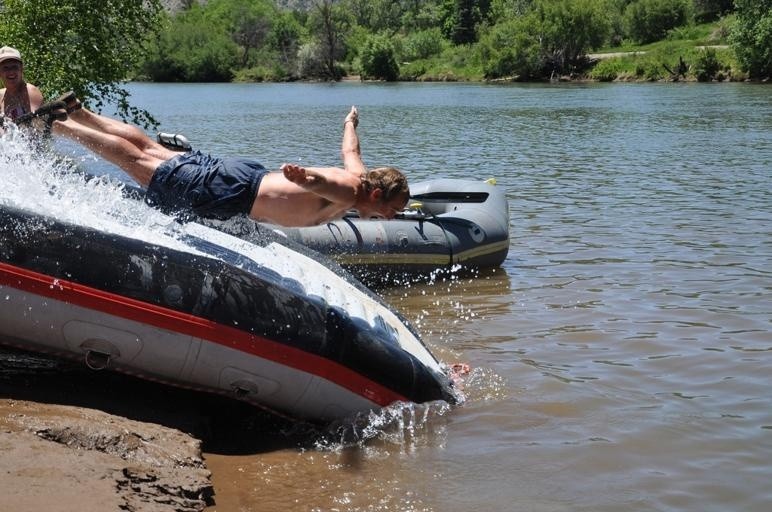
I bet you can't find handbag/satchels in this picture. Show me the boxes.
[343,120,353,127]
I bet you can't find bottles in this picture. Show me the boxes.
[0,45,23,65]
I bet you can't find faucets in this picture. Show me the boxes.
[13,101,70,140]
[56,90,83,115]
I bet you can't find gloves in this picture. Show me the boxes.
[87,175,510,290]
[0,204,460,455]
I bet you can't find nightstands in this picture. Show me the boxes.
[408,192,489,203]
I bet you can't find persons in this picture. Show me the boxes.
[0,44,44,146]
[36,90,409,226]
